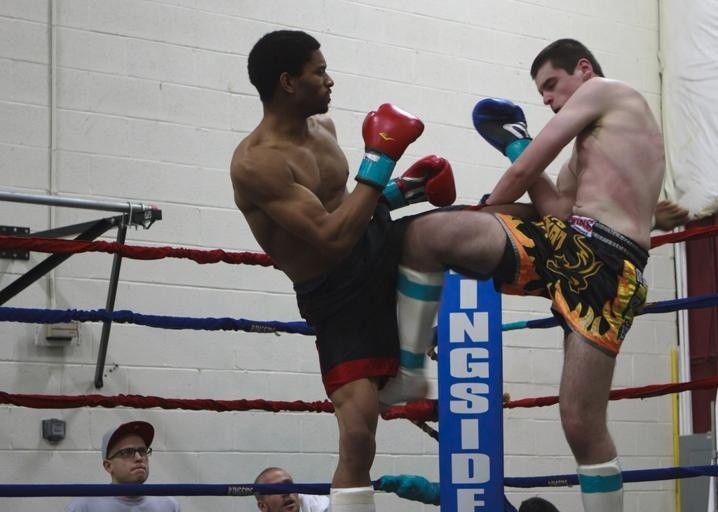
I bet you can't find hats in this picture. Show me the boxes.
[101,421,154,462]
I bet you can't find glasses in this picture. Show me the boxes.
[108,447,152,461]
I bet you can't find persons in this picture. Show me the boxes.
[250,464,303,512]
[369,36,668,512]
[229,31,459,512]
[67,419,185,512]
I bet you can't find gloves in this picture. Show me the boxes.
[472,96,533,163]
[356,102,425,188]
[380,154,456,210]
